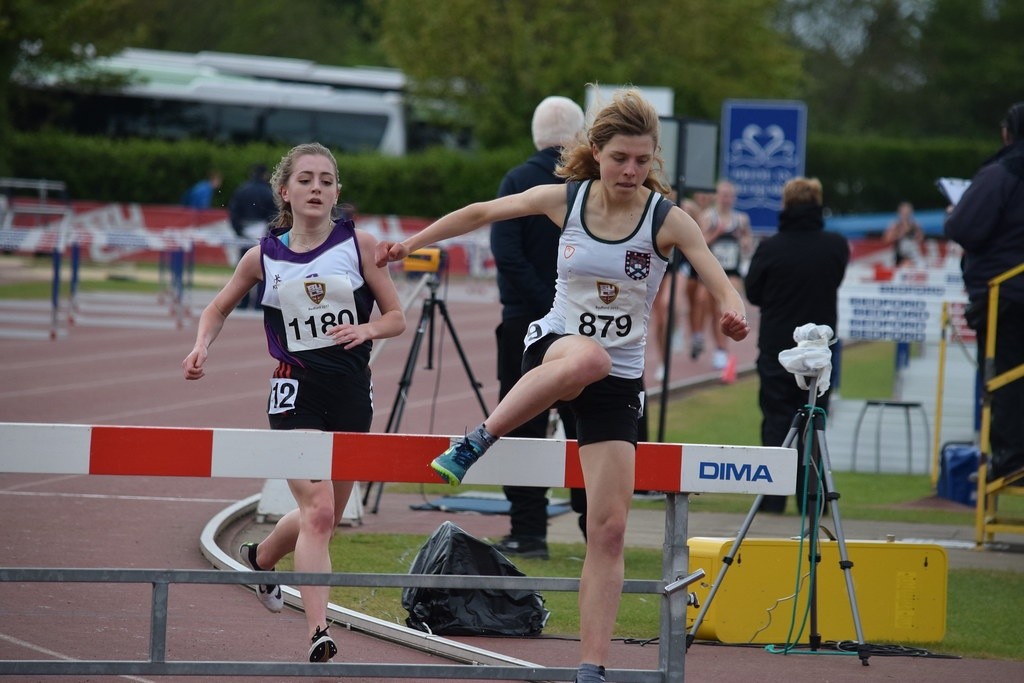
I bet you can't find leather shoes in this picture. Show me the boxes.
[491,536,551,560]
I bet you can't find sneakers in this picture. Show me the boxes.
[308,619,338,663]
[656,366,664,380]
[690,334,704,359]
[431,425,479,487]
[239,541,283,613]
[714,352,728,369]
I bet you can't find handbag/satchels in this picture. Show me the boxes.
[936,440,993,509]
[399,519,550,639]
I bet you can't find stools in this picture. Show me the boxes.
[850,399,930,476]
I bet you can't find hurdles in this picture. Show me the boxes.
[0,231,978,682]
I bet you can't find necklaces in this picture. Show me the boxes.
[290,221,332,252]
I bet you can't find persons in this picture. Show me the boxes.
[228,165,279,309]
[374,94,750,683]
[490,95,587,559]
[744,178,848,513]
[883,202,922,241]
[654,181,749,382]
[182,171,220,210]
[944,104,1024,485]
[180,143,406,665]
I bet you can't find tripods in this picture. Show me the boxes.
[362,275,490,512]
[686,379,872,667]
